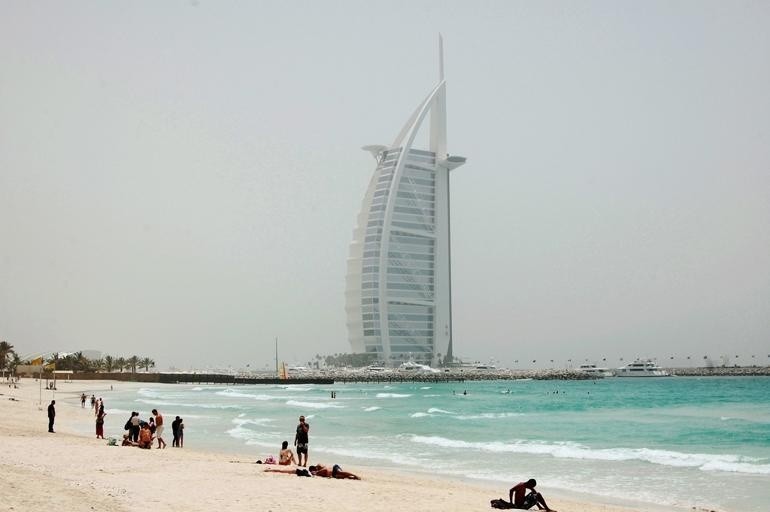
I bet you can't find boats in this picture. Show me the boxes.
[395,357,441,375]
[615,358,672,377]
[573,364,614,378]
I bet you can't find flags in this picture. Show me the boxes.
[45,363,55,370]
[32,356,42,365]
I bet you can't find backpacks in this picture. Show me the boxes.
[125,419,130,430]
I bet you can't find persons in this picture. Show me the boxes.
[48,400,55,432]
[510,479,557,512]
[309,463,361,480]
[81,393,107,439]
[279,441,296,465]
[294,416,309,467]
[122,409,184,449]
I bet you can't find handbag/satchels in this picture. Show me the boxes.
[265,456,275,464]
[151,425,156,433]
[100,412,107,419]
[491,498,513,508]
